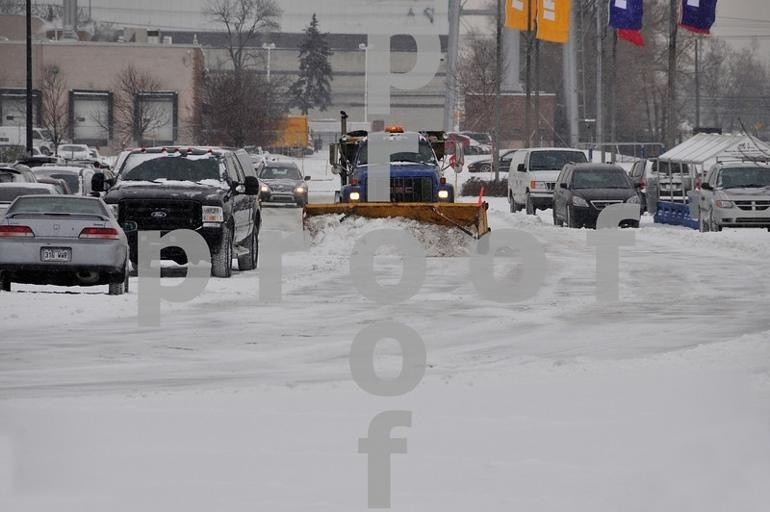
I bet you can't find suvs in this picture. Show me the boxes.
[627,157,694,216]
[242,144,264,168]
[699,161,770,234]
[92,146,263,279]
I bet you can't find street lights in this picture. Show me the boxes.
[358,41,375,122]
[263,41,276,83]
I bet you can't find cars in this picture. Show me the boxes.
[1,194,137,293]
[694,172,708,190]
[444,132,497,155]
[254,156,312,206]
[468,149,518,172]
[0,142,132,218]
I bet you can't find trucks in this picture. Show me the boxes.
[329,109,465,204]
[0,125,55,158]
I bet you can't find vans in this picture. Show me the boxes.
[507,147,587,215]
[552,161,641,229]
[305,134,315,154]
